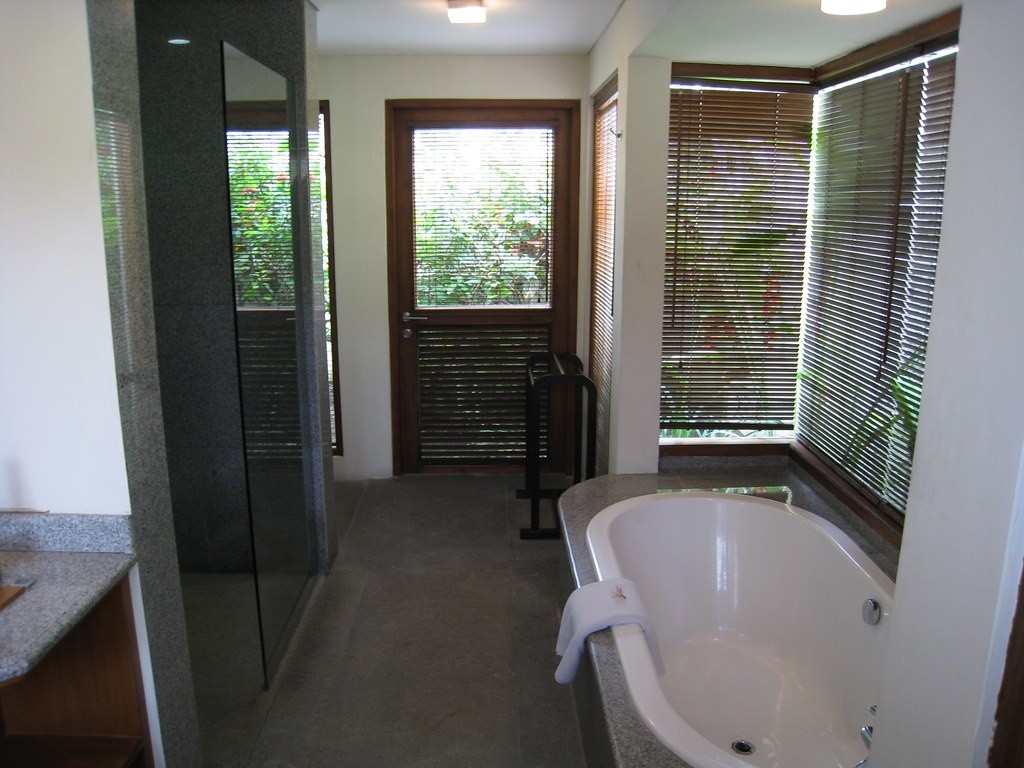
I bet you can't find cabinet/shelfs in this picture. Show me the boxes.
[0,574,155,768]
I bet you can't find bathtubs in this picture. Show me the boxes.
[556,469,897,768]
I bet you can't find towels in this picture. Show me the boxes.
[553,580,666,688]
